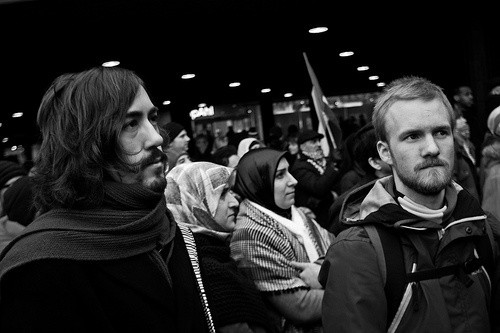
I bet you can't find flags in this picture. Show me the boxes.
[304,53,342,156]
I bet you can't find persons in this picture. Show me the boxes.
[0,67,500,333]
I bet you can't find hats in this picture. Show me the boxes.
[297,130,324,145]
[162,121,185,144]
